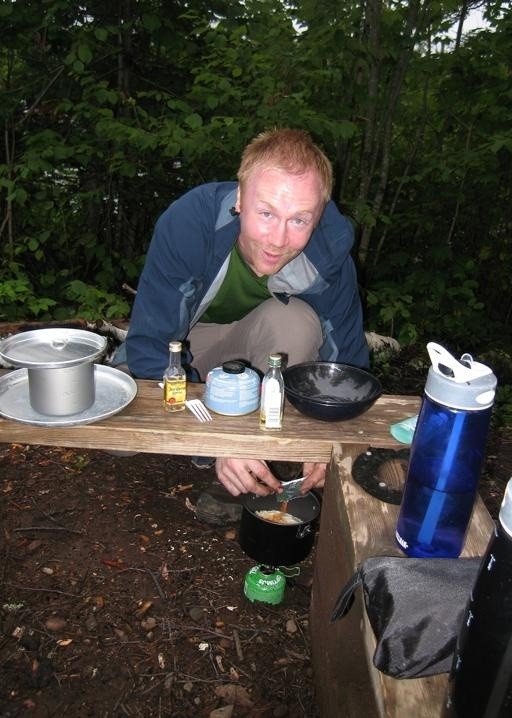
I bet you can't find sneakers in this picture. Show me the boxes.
[195,460,303,528]
[189,456,216,470]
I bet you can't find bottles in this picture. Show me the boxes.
[441,478,511,717]
[257,354,290,432]
[389,414,420,443]
[394,339,501,559]
[162,340,187,413]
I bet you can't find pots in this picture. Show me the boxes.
[237,481,322,564]
[280,359,385,421]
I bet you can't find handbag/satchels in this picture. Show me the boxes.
[328,556,484,680]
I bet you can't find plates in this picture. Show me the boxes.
[0,325,109,366]
[0,363,140,426]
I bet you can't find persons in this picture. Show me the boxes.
[115,127,371,496]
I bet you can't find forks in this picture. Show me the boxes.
[157,380,212,423]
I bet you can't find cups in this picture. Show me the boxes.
[28,361,97,418]
